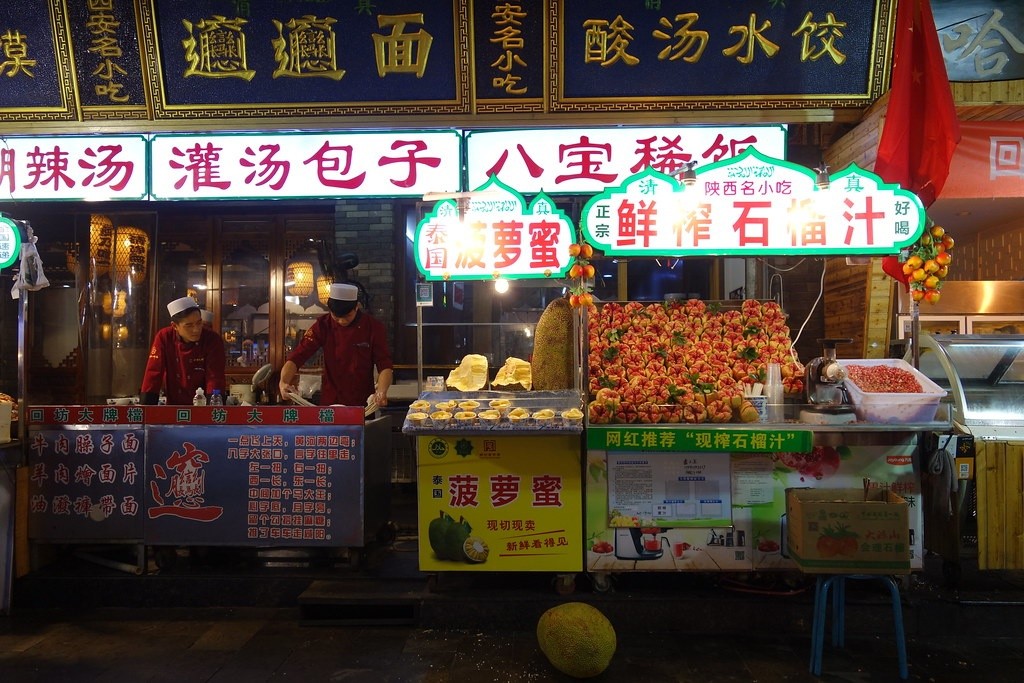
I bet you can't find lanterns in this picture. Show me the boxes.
[288,263,314,298]
[285,324,296,342]
[102,292,113,315]
[101,321,112,342]
[113,291,127,318]
[108,225,150,285]
[187,289,198,304]
[66,248,80,274]
[317,275,334,306]
[88,213,114,278]
[114,323,128,343]
[224,331,236,344]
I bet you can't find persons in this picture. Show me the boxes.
[138,295,227,407]
[279,282,393,420]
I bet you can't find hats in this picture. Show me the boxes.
[167,296,197,317]
[199,309,215,323]
[328,283,358,301]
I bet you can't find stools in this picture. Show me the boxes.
[809,574,908,683]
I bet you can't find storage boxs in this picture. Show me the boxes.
[837,359,949,423]
[788,487,911,573]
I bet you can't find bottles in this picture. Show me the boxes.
[193,386,206,406]
[209,389,223,406]
[226,396,239,405]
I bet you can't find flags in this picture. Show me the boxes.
[871,0,959,291]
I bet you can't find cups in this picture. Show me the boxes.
[745,396,769,422]
[763,363,784,421]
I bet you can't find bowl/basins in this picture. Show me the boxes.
[478,411,501,427]
[135,397,140,403]
[430,411,452,427]
[561,411,584,425]
[107,398,129,406]
[409,403,431,414]
[507,413,530,426]
[457,401,480,411]
[488,401,512,418]
[130,398,135,404]
[453,412,476,426]
[532,412,555,425]
[406,412,428,427]
[435,402,457,413]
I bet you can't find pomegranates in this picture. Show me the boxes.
[846,364,926,394]
[568,244,595,309]
[902,224,954,305]
[777,446,840,480]
[586,298,805,423]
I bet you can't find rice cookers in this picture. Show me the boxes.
[229,364,273,404]
